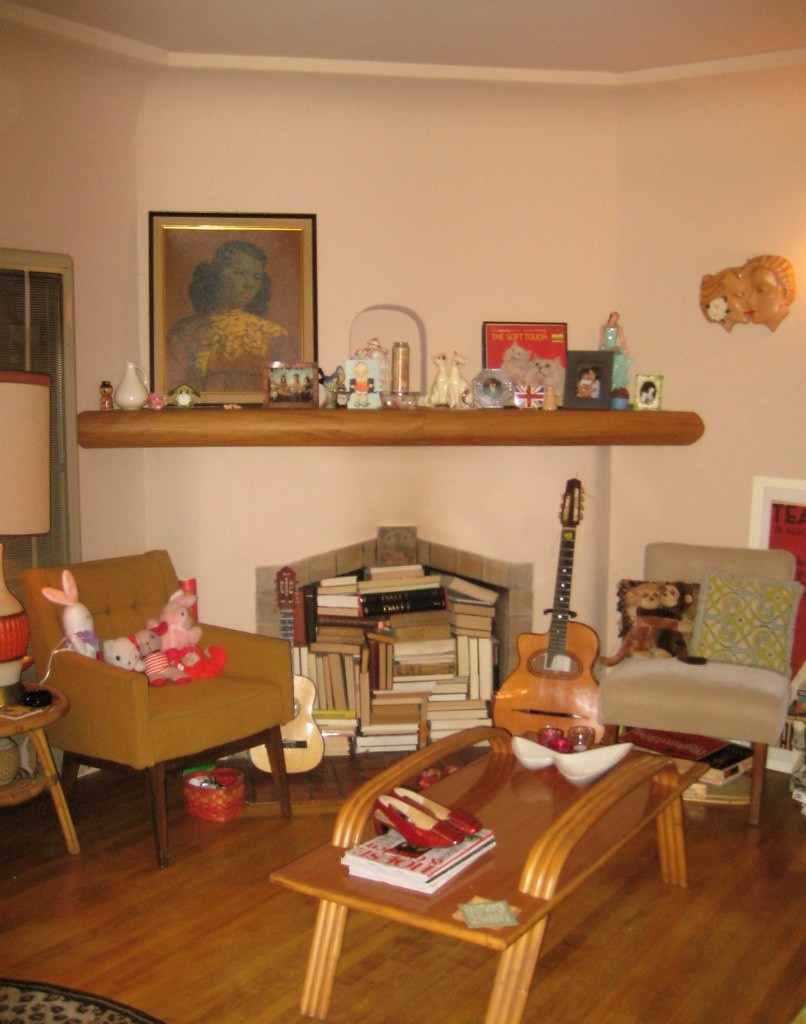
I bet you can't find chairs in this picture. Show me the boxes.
[21,550,294,870]
[596,542,805,826]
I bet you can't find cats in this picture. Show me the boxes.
[499,340,566,407]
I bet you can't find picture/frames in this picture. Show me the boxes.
[563,350,615,410]
[148,211,318,408]
[632,373,664,411]
[261,360,318,408]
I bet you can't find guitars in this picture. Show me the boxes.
[246,564,327,775]
[492,477,620,747]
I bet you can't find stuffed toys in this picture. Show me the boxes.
[595,579,706,665]
[40,569,226,687]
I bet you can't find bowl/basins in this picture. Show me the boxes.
[379,391,421,408]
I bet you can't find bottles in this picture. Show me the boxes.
[115,361,148,411]
[100,381,114,412]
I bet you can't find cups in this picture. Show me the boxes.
[537,726,595,754]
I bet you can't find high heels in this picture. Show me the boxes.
[372,794,464,848]
[392,786,482,834]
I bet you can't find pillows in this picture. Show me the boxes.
[689,565,806,677]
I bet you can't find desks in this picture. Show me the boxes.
[268,725,709,1024]
[0,681,82,856]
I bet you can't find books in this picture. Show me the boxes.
[291,563,500,757]
[618,729,754,805]
[0,703,42,721]
[341,824,496,894]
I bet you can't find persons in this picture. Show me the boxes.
[598,312,632,389]
[278,373,311,400]
[577,367,600,398]
